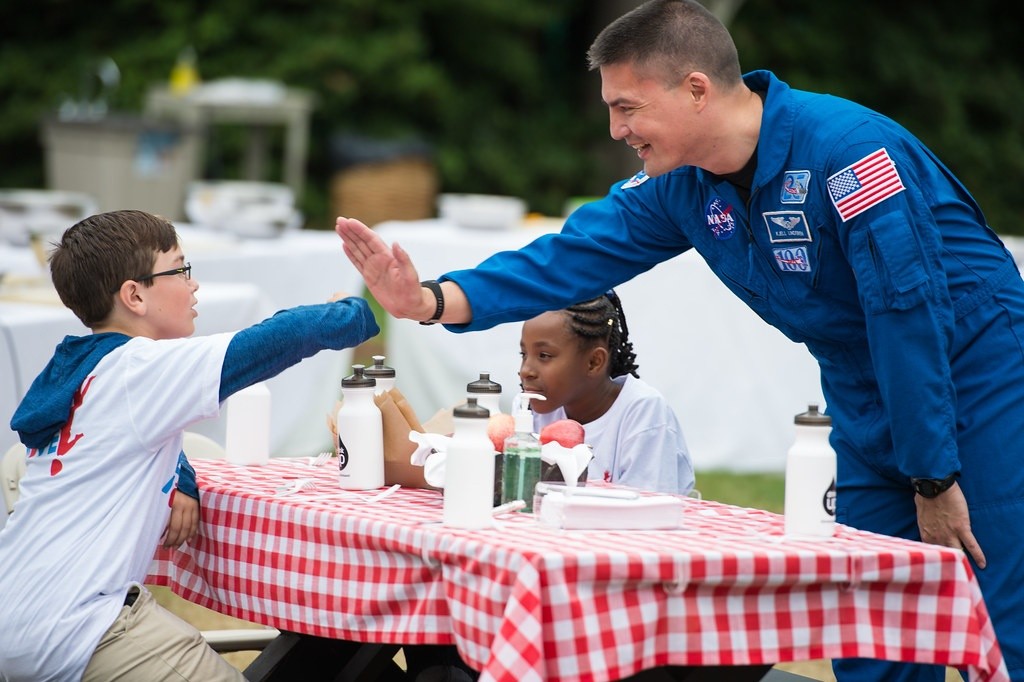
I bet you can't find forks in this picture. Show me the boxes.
[285,479,317,490]
[274,452,333,466]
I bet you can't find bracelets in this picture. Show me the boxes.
[419,279,445,325]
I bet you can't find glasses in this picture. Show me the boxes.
[110,262,192,295]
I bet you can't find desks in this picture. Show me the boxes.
[140,455,1011,682]
[0,218,1023,524]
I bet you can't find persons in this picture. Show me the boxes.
[335,0,1024,682]
[0,208,380,682]
[521,286,700,500]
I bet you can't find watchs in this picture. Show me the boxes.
[911,474,957,500]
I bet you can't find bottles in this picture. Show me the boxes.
[784,404,838,542]
[443,397,496,530]
[466,371,502,418]
[363,356,396,396]
[226,382,271,467]
[336,364,385,490]
